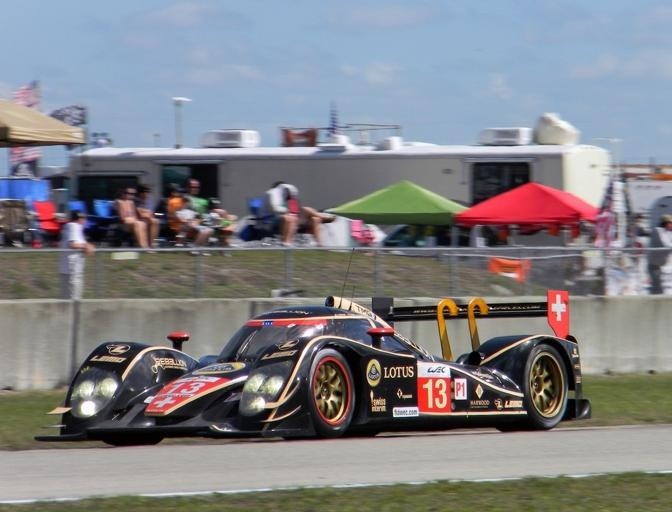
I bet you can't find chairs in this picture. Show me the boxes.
[0,199,278,248]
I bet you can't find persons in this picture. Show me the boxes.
[56,209,96,299]
[648,214,672,294]
[114,176,337,247]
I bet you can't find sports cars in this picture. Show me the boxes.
[33,295,592,445]
